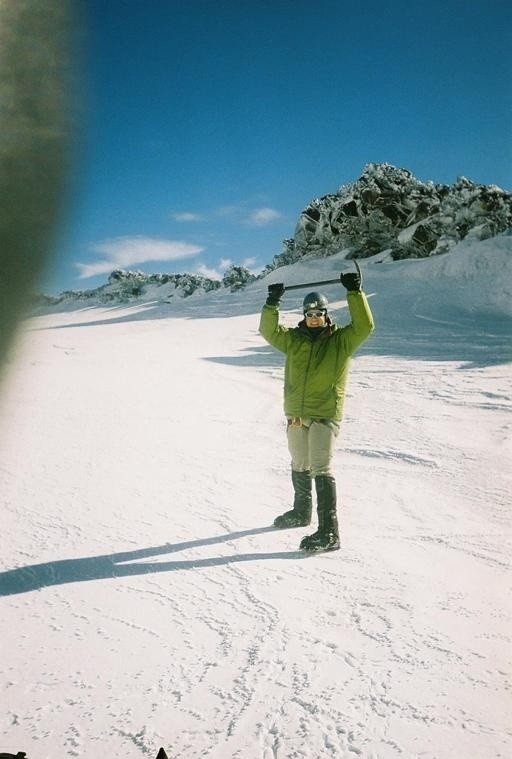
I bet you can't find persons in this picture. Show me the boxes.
[256,273,374,553]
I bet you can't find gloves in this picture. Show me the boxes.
[340,272,360,291]
[266,283,285,305]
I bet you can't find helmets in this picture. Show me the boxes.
[303,291,328,312]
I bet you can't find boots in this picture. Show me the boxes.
[273,470,312,528]
[300,475,340,550]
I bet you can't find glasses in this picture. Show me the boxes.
[305,310,325,318]
